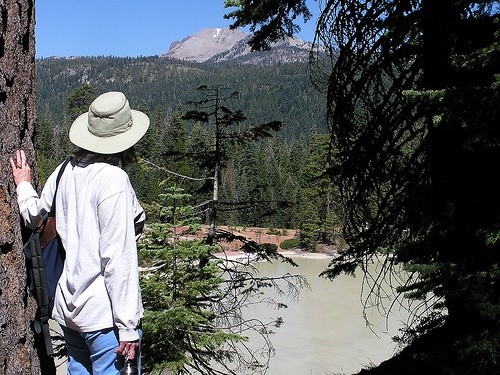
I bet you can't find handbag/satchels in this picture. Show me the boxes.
[26,217,65,301]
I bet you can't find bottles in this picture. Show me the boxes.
[120,353,139,375]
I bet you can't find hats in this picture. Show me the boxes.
[69,91,150,154]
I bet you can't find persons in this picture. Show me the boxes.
[10,91,150,375]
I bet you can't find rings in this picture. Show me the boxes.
[17,165,22,168]
